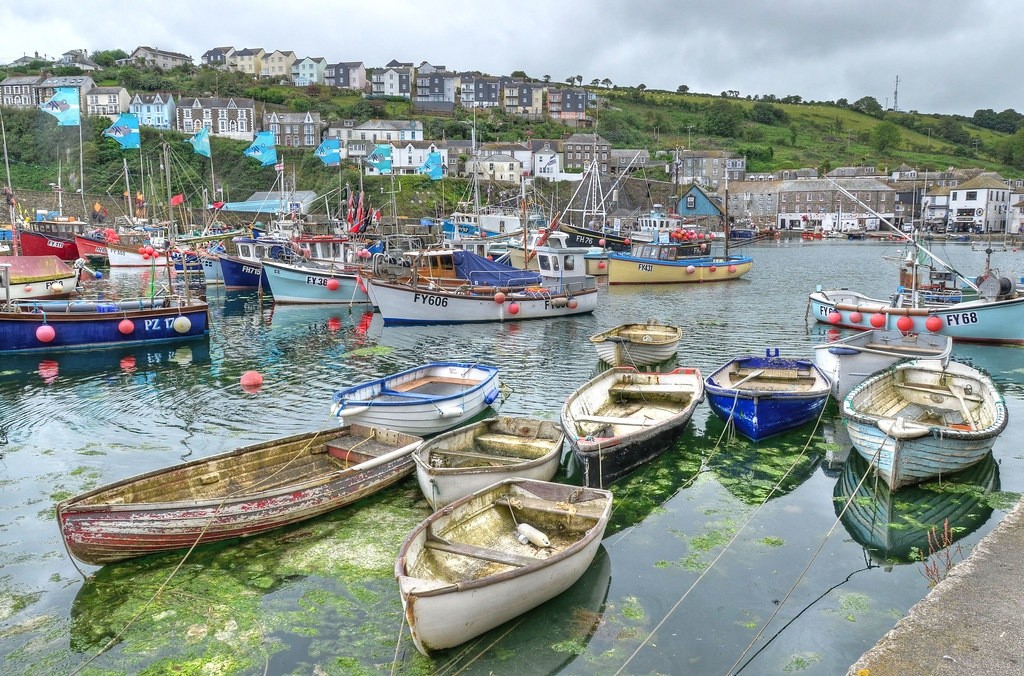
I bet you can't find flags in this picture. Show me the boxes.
[1,186,225,222]
[346,189,381,233]
[275,164,285,170]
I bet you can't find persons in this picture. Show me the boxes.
[73,256,91,287]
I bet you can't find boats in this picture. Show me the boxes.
[0,126,1024,355]
[54,321,1009,660]
